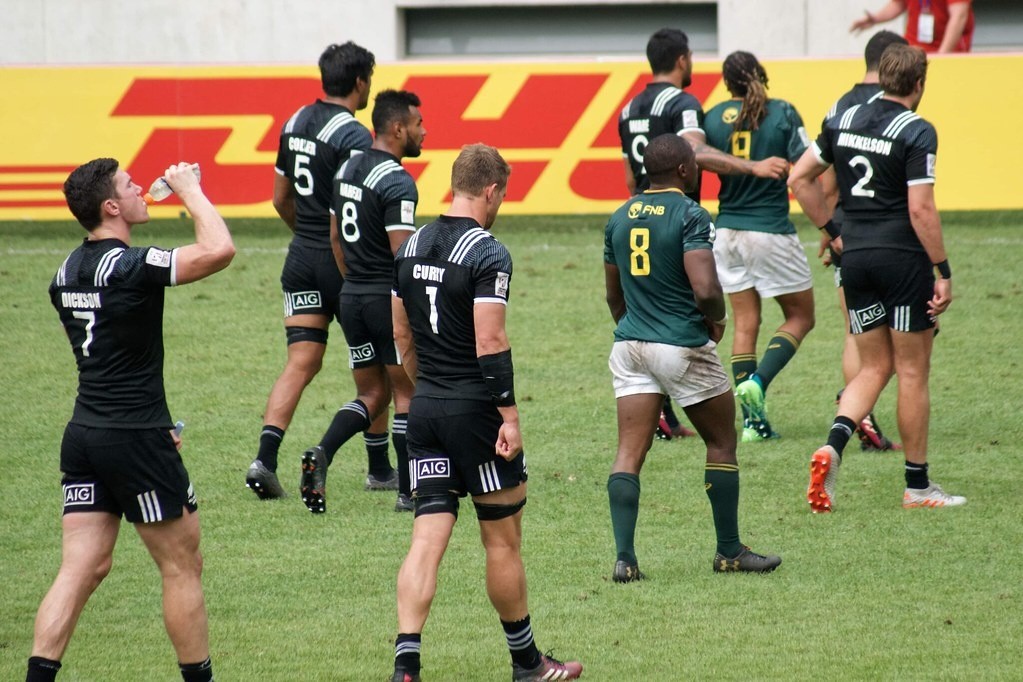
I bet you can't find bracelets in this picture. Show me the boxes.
[932,260,952,280]
[819,218,842,241]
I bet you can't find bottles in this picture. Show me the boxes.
[143,162,201,205]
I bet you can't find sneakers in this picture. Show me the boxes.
[613,562,647,581]
[741,423,782,442]
[836,388,885,450]
[903,479,966,509]
[365,471,400,490]
[246,459,286,500]
[714,544,781,572]
[734,379,771,439]
[670,425,694,438]
[512,648,582,682]
[395,494,415,511]
[654,411,672,441]
[808,445,841,514]
[389,669,421,682]
[299,446,328,513]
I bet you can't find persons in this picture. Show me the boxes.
[787,43,968,515]
[603,133,783,580]
[298,89,427,514]
[818,30,909,452]
[849,0,976,53]
[618,30,788,441]
[22,155,239,682]
[246,40,398,497]
[385,142,583,682]
[699,47,832,439]
